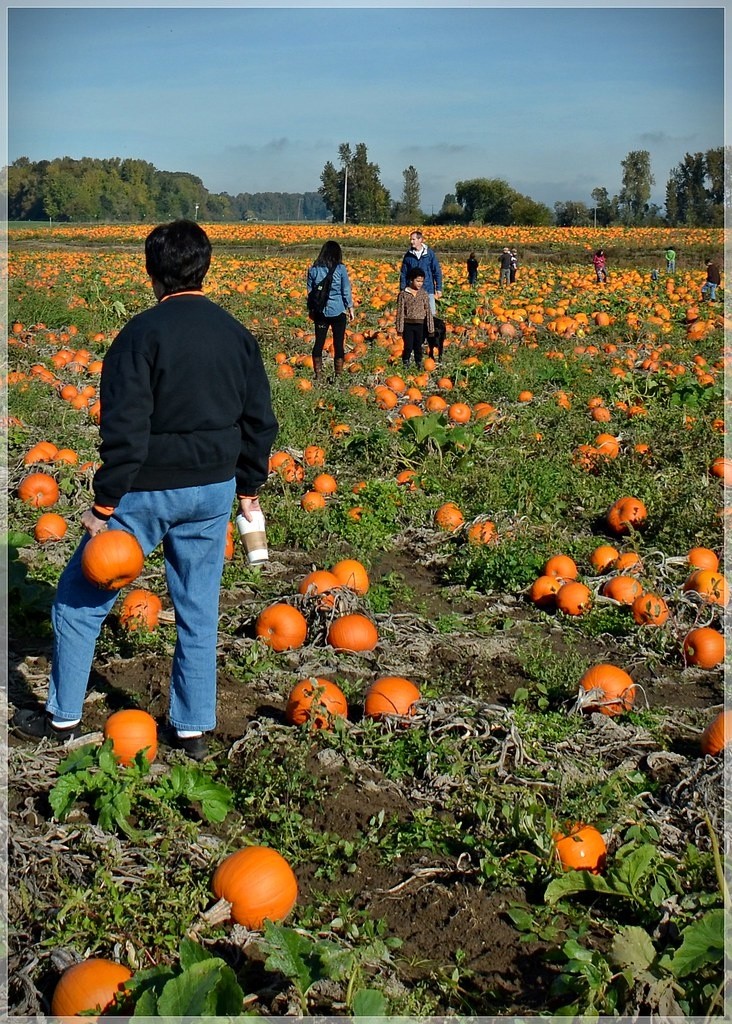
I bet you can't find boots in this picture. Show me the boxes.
[711,299,714,301]
[402,359,409,370]
[333,357,344,380]
[312,355,325,379]
[698,292,705,302]
[416,361,421,372]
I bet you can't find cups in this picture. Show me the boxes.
[235,510,269,566]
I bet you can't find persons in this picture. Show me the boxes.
[466,253,479,284]
[592,249,608,283]
[396,232,442,371]
[652,267,659,281]
[665,246,676,274]
[306,240,354,388]
[698,259,721,303]
[11,220,279,760]
[497,248,518,289]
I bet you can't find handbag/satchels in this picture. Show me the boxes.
[307,262,337,315]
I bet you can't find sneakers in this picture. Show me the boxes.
[156,726,209,760]
[10,707,83,745]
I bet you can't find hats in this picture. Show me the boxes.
[502,248,509,250]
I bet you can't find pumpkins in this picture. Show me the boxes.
[120,590,161,635]
[201,254,732,754]
[7,224,157,238]
[1,252,153,588]
[196,223,731,250]
[52,960,133,1023]
[551,821,608,876]
[105,710,157,766]
[213,845,298,927]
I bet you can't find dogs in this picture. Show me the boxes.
[423,315,447,364]
